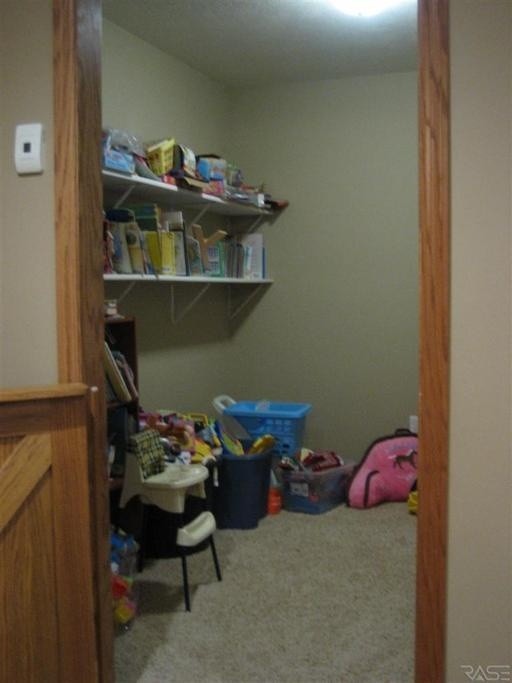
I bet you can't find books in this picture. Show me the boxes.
[113,347,139,399]
[103,200,268,279]
[103,340,133,402]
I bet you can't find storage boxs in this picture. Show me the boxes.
[281,456,362,514]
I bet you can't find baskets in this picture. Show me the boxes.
[212,395,312,458]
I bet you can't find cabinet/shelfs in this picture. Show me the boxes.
[103,316,141,486]
[97,165,276,321]
